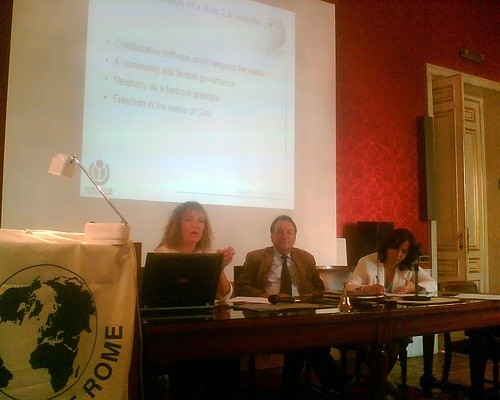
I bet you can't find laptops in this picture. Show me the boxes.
[141,252,224,315]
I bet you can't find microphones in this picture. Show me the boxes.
[268,294,301,304]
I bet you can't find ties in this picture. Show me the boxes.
[280,255,292,296]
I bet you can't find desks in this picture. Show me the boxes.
[143,291,500,400]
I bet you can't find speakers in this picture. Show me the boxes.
[357,221,394,257]
[423,116,437,221]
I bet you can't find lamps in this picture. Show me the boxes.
[48,154,130,245]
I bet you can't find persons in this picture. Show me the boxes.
[154,200,236,400]
[344,228,437,400]
[237,215,369,400]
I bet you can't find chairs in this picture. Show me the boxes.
[438,280,500,393]
[234,265,325,394]
